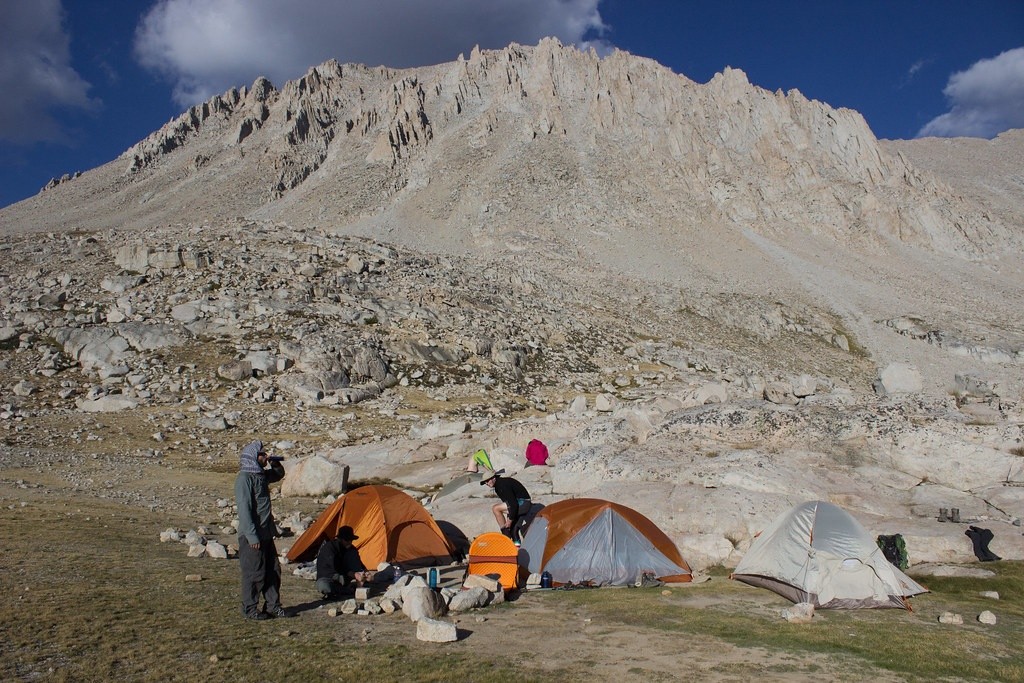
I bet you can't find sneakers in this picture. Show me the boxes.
[240,607,268,620]
[262,608,293,617]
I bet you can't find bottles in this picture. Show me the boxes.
[541,571,552,587]
[393,567,401,583]
[429,568,437,591]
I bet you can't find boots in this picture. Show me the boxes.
[938,507,948,521]
[951,508,960,523]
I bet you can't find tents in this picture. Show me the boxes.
[729,499,929,613]
[517,498,693,586]
[285,485,457,570]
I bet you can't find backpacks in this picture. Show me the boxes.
[877,533,909,571]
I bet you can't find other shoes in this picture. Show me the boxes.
[514,540,520,546]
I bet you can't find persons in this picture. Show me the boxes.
[234,440,285,621]
[315,525,374,598]
[524,438,548,469]
[480,471,545,549]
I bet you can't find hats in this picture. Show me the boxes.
[479,471,501,485]
[333,526,359,540]
